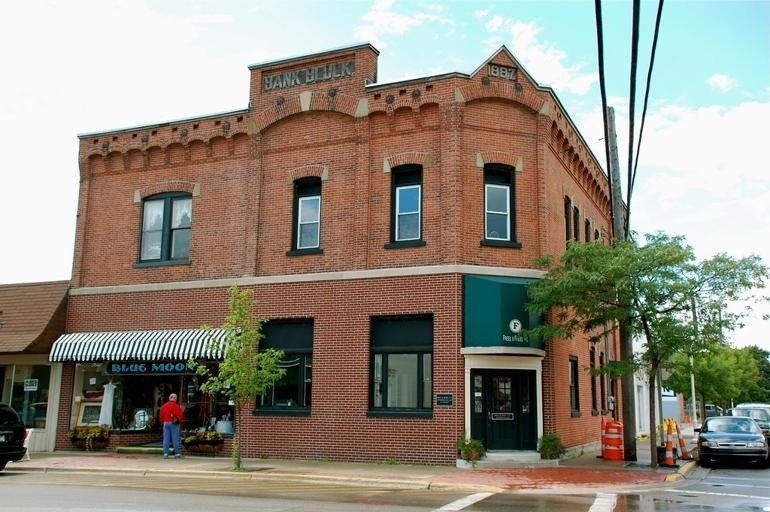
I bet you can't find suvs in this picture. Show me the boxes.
[0,403,28,473]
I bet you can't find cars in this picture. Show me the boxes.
[694,403,725,418]
[695,416,769,466]
[729,404,770,439]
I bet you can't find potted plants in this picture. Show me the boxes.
[457,433,487,468]
[67,426,108,451]
[175,432,224,456]
[538,434,566,461]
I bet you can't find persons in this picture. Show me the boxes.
[160,392,183,459]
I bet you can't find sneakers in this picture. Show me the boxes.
[164,456,182,459]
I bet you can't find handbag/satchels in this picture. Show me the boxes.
[172,416,178,424]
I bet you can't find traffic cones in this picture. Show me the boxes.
[660,423,680,468]
[672,420,694,461]
[596,417,606,458]
